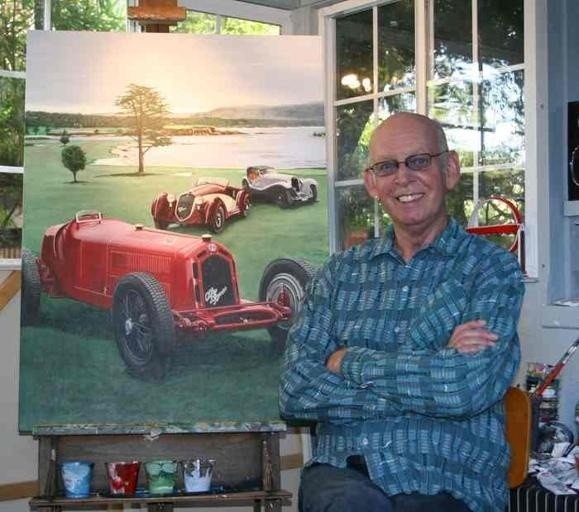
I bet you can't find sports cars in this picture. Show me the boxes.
[152,175,250,232]
[243,166,319,209]
[22,207,319,382]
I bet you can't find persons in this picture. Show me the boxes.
[277,112,526,512]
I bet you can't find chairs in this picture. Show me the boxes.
[503,384,535,487]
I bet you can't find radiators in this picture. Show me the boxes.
[507,474,579,512]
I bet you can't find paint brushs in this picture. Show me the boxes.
[529,339,579,401]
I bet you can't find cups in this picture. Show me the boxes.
[526,360,559,427]
[104,460,141,498]
[58,460,95,500]
[180,458,215,494]
[144,458,176,495]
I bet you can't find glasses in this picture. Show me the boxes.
[364,151,447,177]
